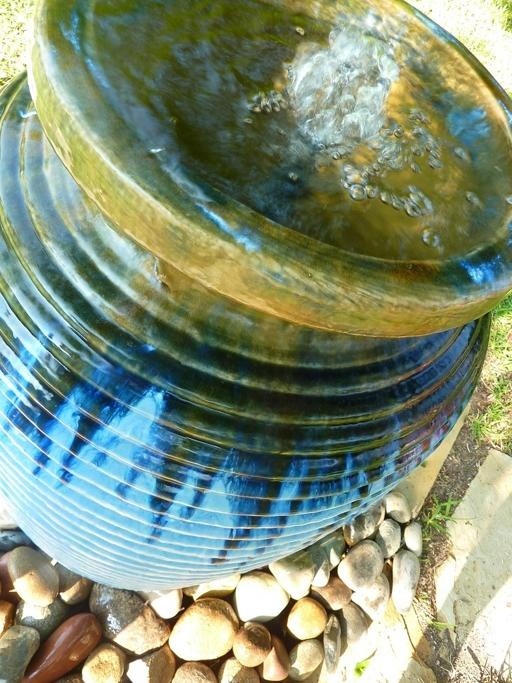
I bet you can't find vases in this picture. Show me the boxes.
[0,1,512,602]
